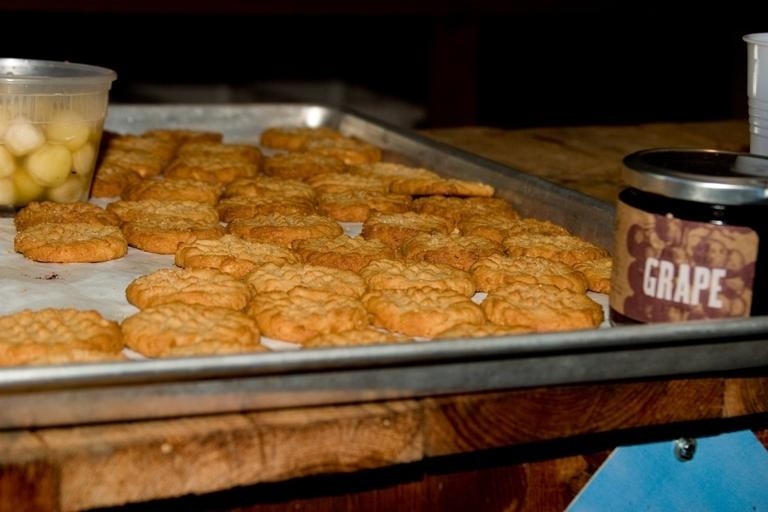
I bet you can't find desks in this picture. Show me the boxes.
[0,117,768,511]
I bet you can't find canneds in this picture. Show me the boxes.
[609,146,768,328]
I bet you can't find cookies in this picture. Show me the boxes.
[0,125,614,370]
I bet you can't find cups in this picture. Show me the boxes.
[0,58,118,218]
[742,33,767,158]
